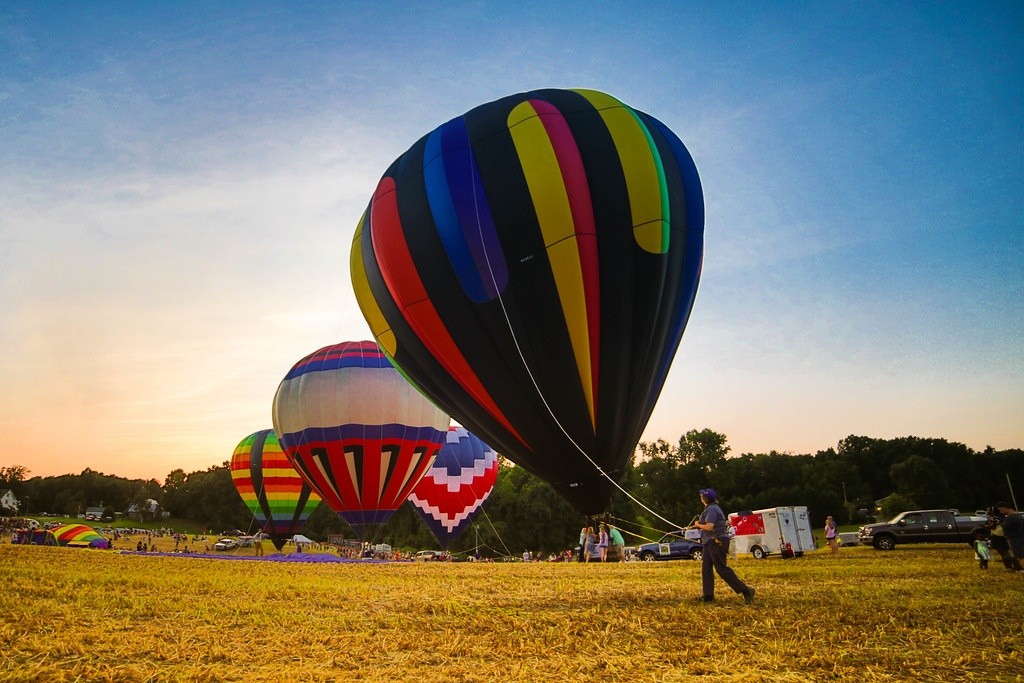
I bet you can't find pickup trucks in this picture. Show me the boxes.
[858,509,991,550]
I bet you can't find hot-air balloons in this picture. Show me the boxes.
[273,340,451,559]
[11,524,108,549]
[405,426,499,560]
[350,88,704,562]
[231,429,323,554]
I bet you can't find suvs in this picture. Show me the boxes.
[215,539,237,550]
[635,530,703,562]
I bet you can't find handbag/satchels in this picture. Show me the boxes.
[991,525,1003,536]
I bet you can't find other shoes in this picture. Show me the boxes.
[695,595,716,604]
[745,587,755,605]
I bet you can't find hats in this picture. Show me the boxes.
[699,489,716,499]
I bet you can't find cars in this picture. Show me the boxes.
[85,515,101,521]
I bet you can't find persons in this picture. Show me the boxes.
[12,529,29,544]
[585,526,594,563]
[523,548,572,562]
[295,541,452,563]
[989,506,1012,568]
[998,502,1024,570]
[480,556,495,563]
[687,489,755,605]
[253,528,263,556]
[578,528,587,562]
[108,528,212,554]
[827,516,837,551]
[599,528,609,562]
[610,526,625,564]
[825,520,837,556]
[974,533,990,569]
